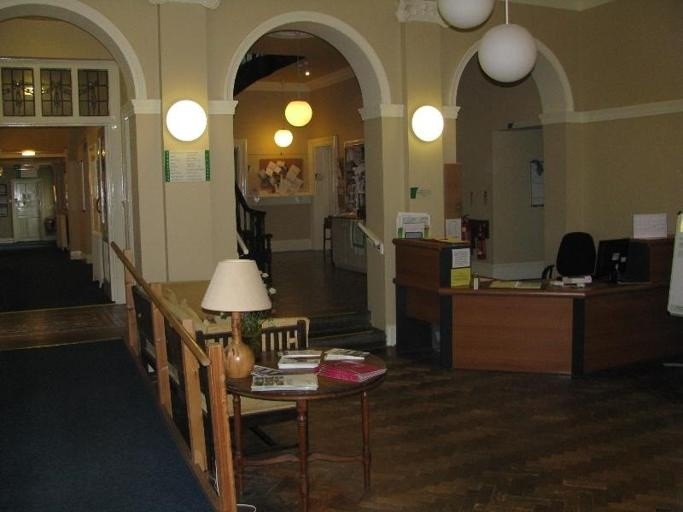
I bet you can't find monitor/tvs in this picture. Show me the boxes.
[595,238,652,287]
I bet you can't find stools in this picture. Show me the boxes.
[323,216,331,262]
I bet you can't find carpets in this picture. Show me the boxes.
[0,337,215,512]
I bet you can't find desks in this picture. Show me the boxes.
[215,347,389,512]
[438,280,683,377]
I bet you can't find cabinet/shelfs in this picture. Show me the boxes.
[598,236,675,283]
[392,236,500,370]
[331,138,368,276]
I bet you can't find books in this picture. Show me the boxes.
[248,347,389,393]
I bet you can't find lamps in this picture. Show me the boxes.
[285,29,313,128]
[478,0,539,83]
[166,98,207,142]
[21,149,36,157]
[438,0,496,32]
[201,258,273,380]
[408,104,444,142]
[274,67,293,148]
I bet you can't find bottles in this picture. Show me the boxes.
[471,272,480,289]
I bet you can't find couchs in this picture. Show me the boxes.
[130,279,310,456]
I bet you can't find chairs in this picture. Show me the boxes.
[540,231,596,281]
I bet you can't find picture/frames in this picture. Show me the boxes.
[529,159,544,208]
